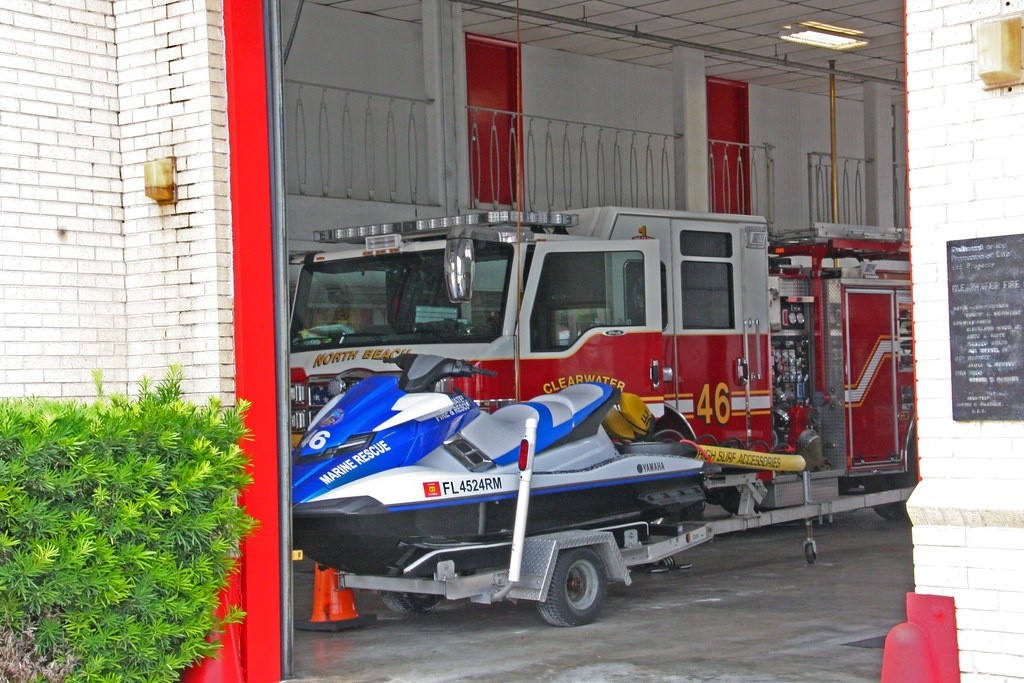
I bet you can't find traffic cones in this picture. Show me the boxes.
[294,560,377,635]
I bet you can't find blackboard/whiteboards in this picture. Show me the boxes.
[943,235,1024,424]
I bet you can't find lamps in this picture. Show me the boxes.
[778,24,870,51]
[977,10,1024,91]
[144,156,178,206]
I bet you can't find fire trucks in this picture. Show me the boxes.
[288,206,917,522]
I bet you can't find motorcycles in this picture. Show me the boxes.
[291,352,706,574]
